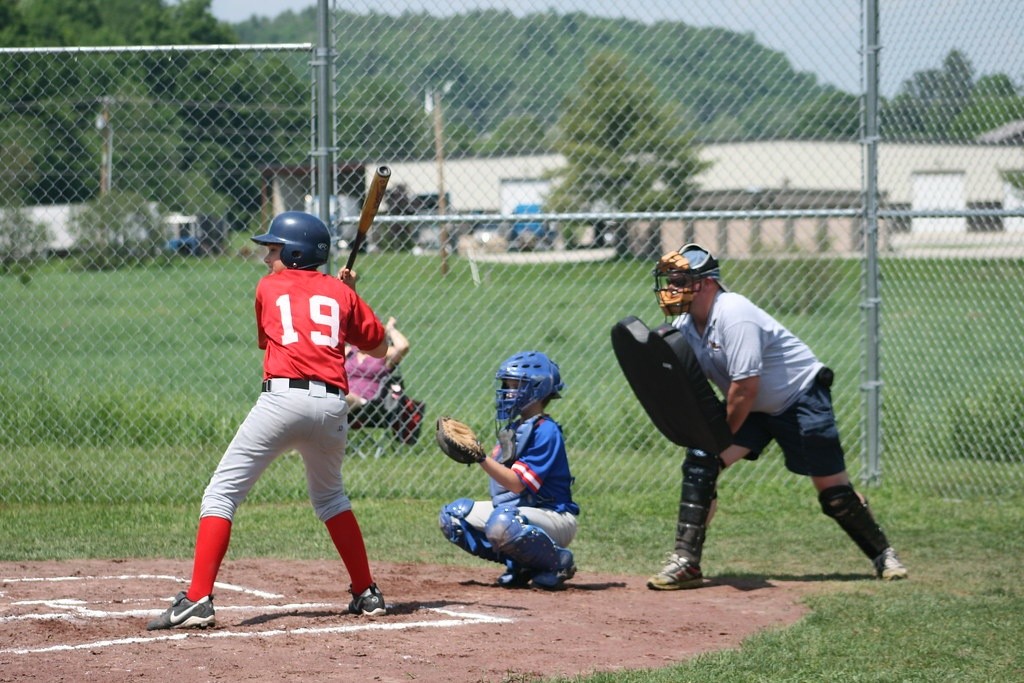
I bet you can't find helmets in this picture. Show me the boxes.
[495,350,563,421]
[250,211,330,269]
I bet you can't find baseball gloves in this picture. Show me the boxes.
[435,416,485,464]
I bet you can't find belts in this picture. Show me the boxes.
[262,379,340,395]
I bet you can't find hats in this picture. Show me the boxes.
[670,249,727,292]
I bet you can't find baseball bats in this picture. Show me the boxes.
[341,164,391,280]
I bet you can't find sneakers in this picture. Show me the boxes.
[498,568,530,583]
[528,564,576,588]
[147,590,216,631]
[347,583,387,616]
[647,552,702,590]
[873,548,906,580]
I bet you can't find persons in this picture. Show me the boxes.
[143,210,389,632]
[344,316,410,415]
[438,348,581,590]
[646,240,910,592]
